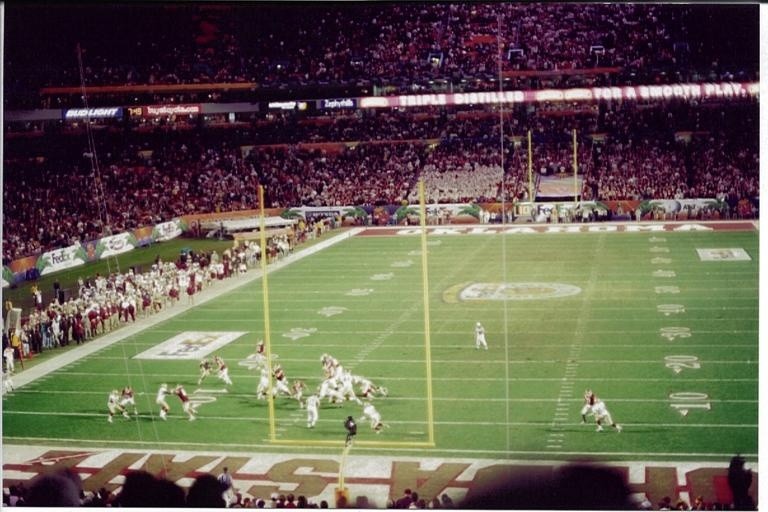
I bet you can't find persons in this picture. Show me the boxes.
[0,0,759,511]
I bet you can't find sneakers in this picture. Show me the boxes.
[579,419,623,433]
[107,410,198,423]
